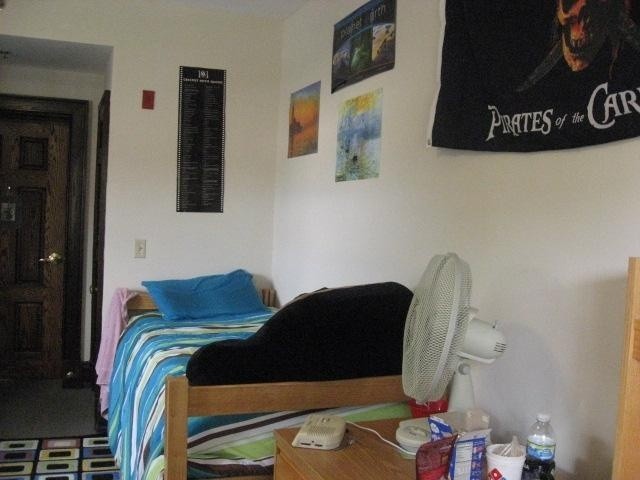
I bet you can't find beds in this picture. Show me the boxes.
[94,272,447,478]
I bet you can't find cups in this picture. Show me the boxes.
[484,443,526,480]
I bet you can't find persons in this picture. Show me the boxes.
[510,1,640,91]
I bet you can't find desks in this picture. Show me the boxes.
[273,419,421,478]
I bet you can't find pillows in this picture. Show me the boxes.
[138,270,273,325]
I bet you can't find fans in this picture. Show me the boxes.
[395,252,506,454]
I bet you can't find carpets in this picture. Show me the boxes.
[1,437,119,480]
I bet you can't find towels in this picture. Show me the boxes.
[95,288,137,423]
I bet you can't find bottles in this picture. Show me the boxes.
[524,411,557,480]
